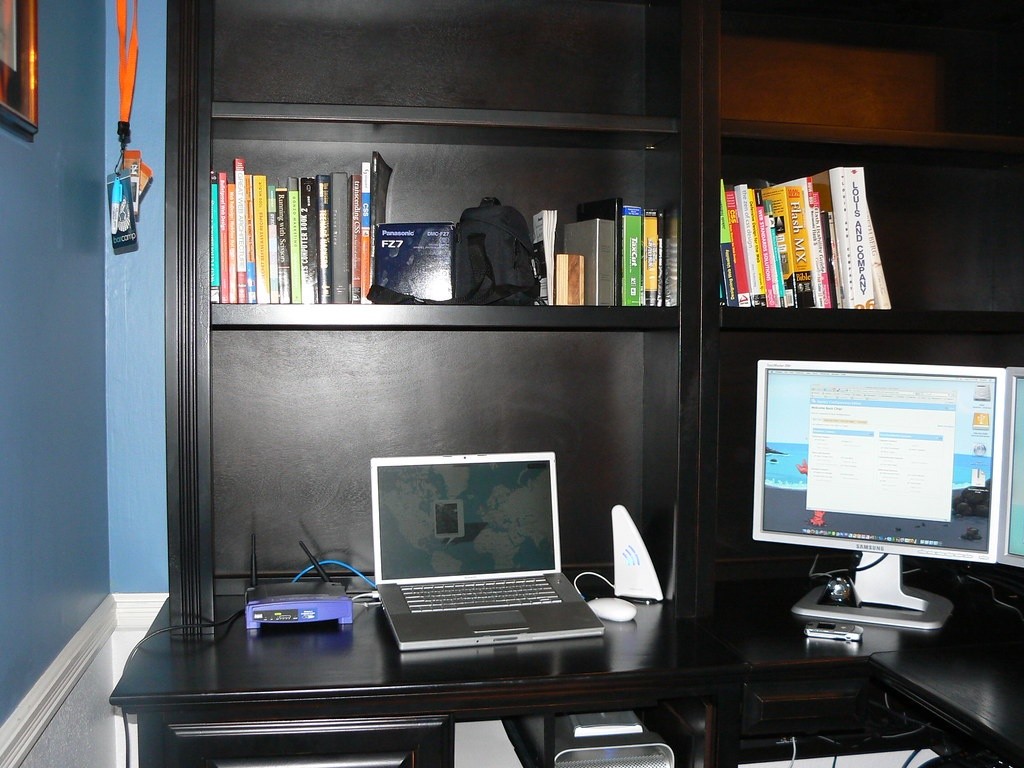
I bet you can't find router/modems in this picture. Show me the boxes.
[245,533,353,629]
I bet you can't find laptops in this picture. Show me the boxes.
[371,452,605,651]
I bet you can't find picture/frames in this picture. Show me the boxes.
[0,0,40,142]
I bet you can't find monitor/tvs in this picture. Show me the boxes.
[997,366,1024,567]
[752,359,1005,630]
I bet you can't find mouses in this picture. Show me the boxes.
[586,597,636,622]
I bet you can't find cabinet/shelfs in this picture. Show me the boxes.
[165,0,1024,636]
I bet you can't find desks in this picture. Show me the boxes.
[109,560,1024,768]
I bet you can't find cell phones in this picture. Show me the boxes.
[804,621,863,640]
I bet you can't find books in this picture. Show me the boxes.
[210,149,665,306]
[719,167,891,310]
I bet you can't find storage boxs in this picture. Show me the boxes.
[374,198,664,306]
